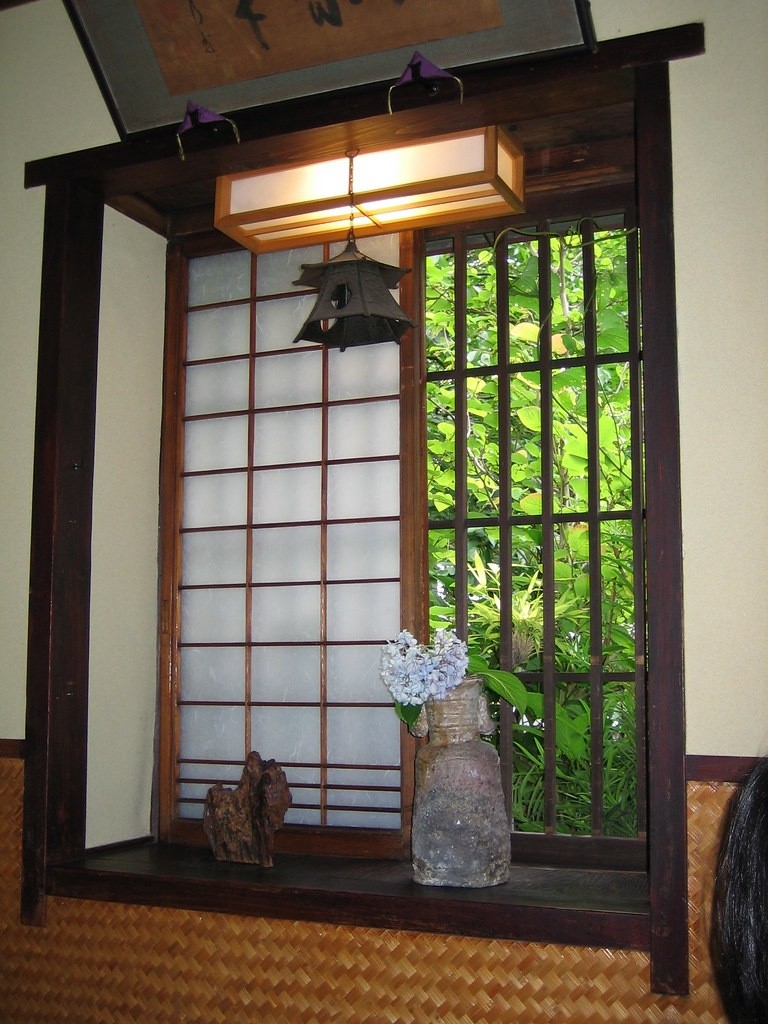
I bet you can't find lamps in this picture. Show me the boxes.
[213,126,527,255]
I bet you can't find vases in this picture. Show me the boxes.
[405,679,511,887]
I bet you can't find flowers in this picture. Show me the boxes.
[380,628,527,729]
